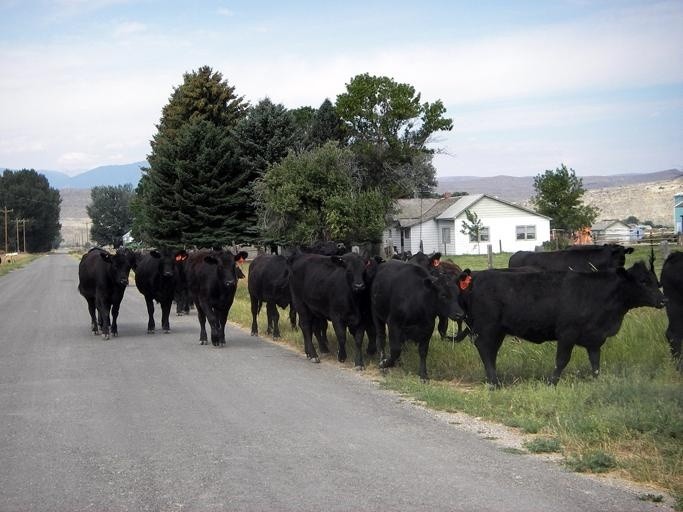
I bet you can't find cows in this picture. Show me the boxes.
[448,262,668,389]
[248,242,473,384]
[647,243,681,368]
[77,246,141,340]
[508,245,634,279]
[133,243,248,346]
